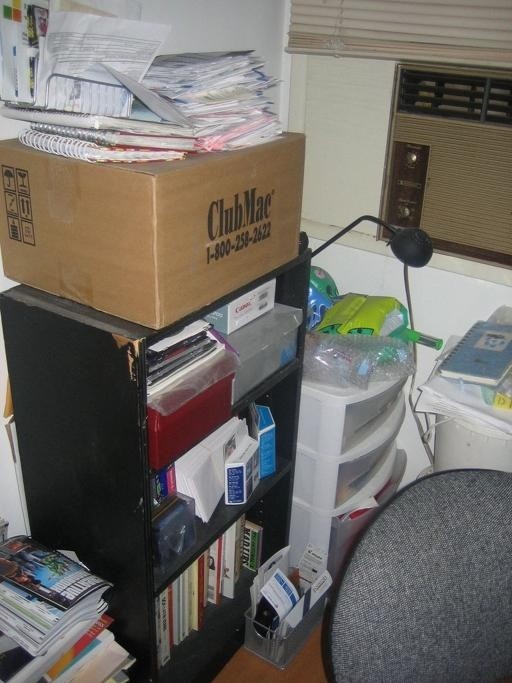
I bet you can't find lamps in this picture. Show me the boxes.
[290,212,431,266]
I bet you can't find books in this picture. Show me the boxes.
[414,304,512,439]
[155,512,262,669]
[146,319,226,402]
[0,535,139,683]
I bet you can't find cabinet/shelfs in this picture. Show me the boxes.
[0,225,310,682]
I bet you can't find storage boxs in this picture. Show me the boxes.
[0,132,308,326]
[286,342,412,611]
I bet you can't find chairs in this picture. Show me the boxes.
[317,469,510,681]
